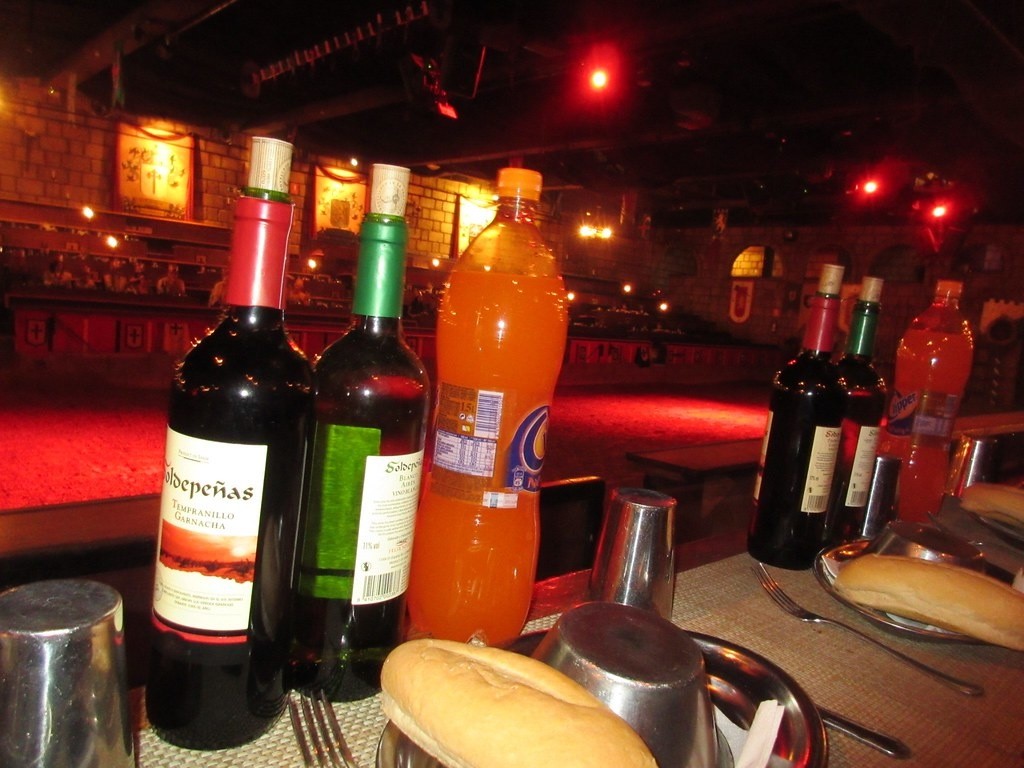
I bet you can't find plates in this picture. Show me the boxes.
[813,539,1013,646]
[374,629,831,768]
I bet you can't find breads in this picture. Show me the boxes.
[833,553,1024,651]
[380,638,659,768]
[958,482,1024,528]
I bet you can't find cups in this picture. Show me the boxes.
[584,487,679,624]
[862,454,902,539]
[0,581,136,768]
[531,601,724,768]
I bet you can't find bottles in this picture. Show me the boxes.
[399,166,568,647]
[290,162,432,704]
[819,277,889,547]
[141,135,319,750]
[886,280,976,527]
[747,263,852,570]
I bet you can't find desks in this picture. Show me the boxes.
[124,524,1024,768]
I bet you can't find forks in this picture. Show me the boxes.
[287,689,359,768]
[750,563,985,697]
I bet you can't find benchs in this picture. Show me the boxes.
[0,460,605,601]
[622,436,765,538]
